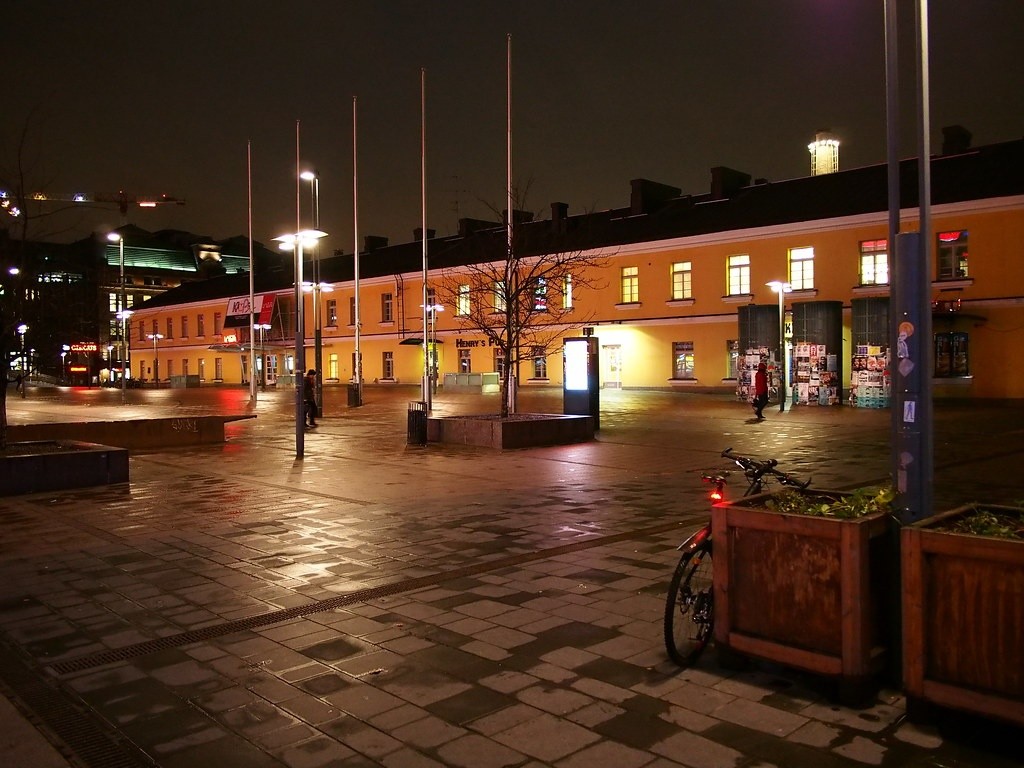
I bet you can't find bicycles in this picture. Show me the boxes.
[662,447,814,663]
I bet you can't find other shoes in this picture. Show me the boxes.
[755,412,766,419]
[304,422,317,429]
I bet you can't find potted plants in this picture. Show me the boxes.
[710,486,899,708]
[325,378,340,383]
[527,377,550,383]
[668,378,697,383]
[379,378,394,383]
[900,503,1024,725]
[721,377,737,384]
[349,379,364,383]
[214,378,223,383]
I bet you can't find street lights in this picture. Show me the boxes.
[107,346,114,387]
[765,281,793,411]
[148,334,164,389]
[254,324,272,391]
[300,172,323,419]
[18,325,29,398]
[272,228,329,460]
[418,304,445,394]
[107,233,127,406]
[61,352,67,386]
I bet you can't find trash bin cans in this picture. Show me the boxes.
[407,400,427,444]
[347,383,359,407]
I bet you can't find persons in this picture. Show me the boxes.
[759,346,774,371]
[740,372,749,383]
[795,345,824,358]
[820,357,826,368]
[853,357,885,369]
[809,387,818,402]
[819,372,837,396]
[755,363,768,418]
[302,369,317,428]
[15,372,21,390]
